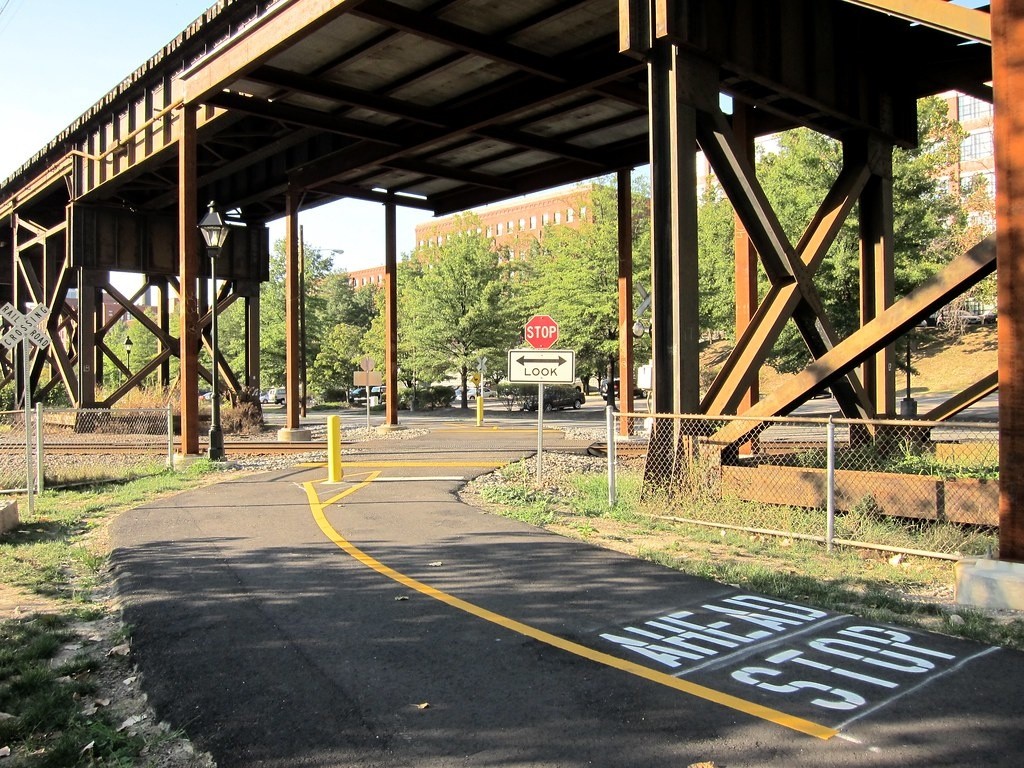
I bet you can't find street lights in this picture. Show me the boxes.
[195,200,233,462]
[123,335,134,401]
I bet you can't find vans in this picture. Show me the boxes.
[268,388,303,406]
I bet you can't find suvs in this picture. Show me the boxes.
[600,380,648,401]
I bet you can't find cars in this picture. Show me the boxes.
[259,388,272,403]
[544,378,583,393]
[371,386,387,393]
[455,388,472,401]
[457,387,497,400]
[523,386,585,413]
[976,305,998,324]
[936,310,983,326]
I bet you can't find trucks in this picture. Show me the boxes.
[637,365,652,396]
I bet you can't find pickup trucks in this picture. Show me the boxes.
[349,387,383,399]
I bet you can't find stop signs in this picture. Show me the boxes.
[525,314,559,349]
